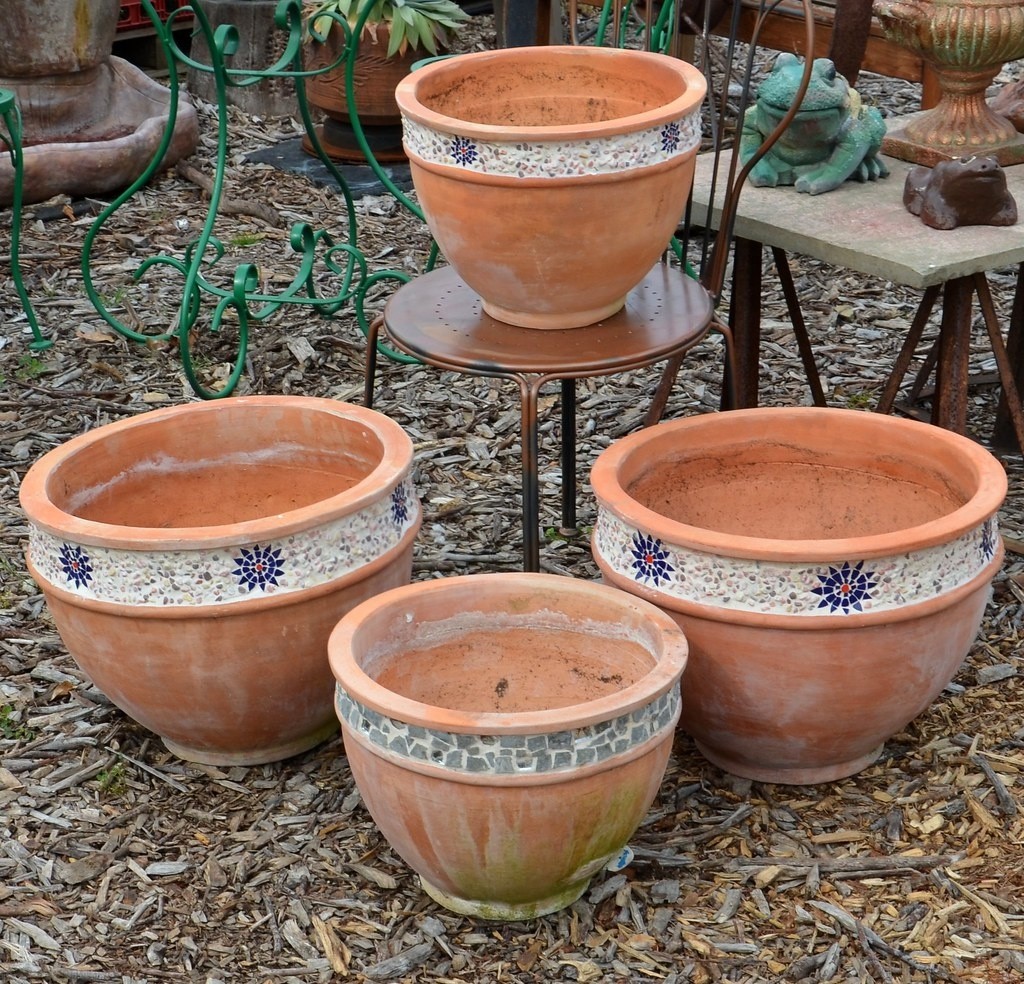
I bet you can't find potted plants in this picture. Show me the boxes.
[302,0,473,164]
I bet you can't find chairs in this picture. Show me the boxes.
[362,1,813,573]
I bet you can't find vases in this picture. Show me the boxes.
[328,572,689,919]
[395,45,707,330]
[18,396,423,767]
[592,405,1009,782]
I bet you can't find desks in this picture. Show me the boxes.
[681,109,1024,460]
